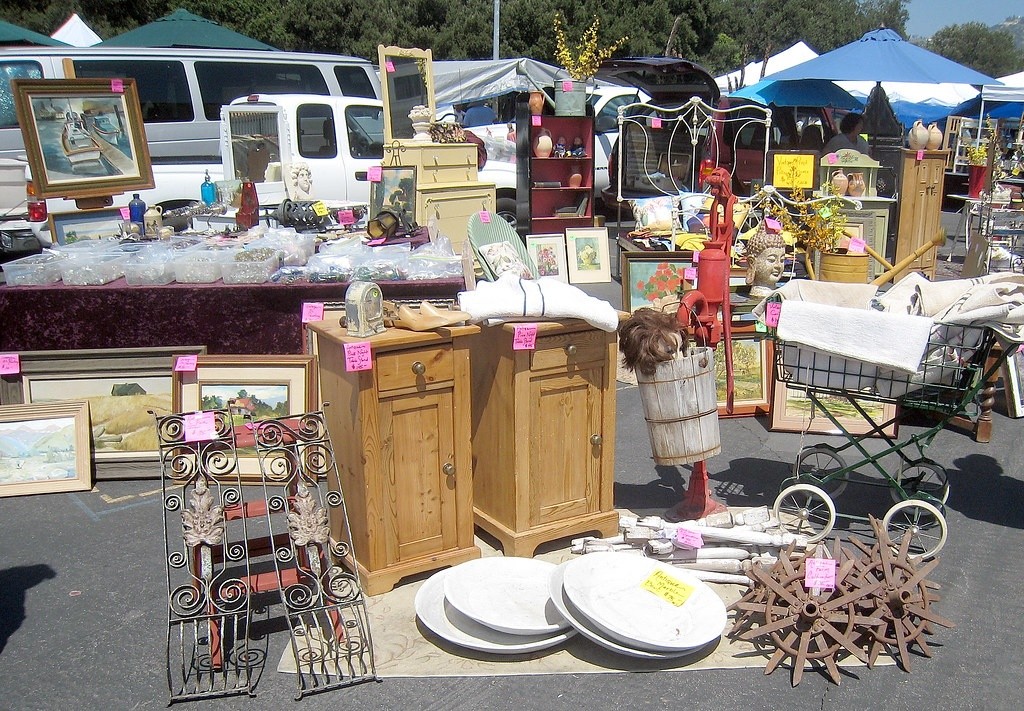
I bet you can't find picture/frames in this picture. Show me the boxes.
[370,165,417,228]
[47,205,129,247]
[768,351,901,440]
[0,400,91,498]
[814,210,875,284]
[171,354,318,487]
[620,251,695,315]
[302,298,457,478]
[1001,348,1024,418]
[687,322,774,419]
[0,344,207,479]
[11,78,157,201]
[526,233,569,285]
[566,227,612,284]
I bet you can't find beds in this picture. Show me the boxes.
[616,96,811,280]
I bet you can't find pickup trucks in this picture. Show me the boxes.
[0,92,384,252]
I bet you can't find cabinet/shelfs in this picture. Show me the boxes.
[470,309,632,560]
[811,148,897,276]
[382,142,497,257]
[307,318,483,598]
[515,90,596,252]
[891,148,950,284]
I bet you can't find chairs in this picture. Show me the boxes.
[598,116,618,129]
[319,119,337,157]
[743,177,778,247]
[799,126,823,150]
[467,212,542,283]
[750,124,780,150]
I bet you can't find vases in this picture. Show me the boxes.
[554,81,586,117]
[564,165,582,187]
[533,127,552,159]
[967,164,987,198]
[819,248,870,283]
[925,122,942,150]
[846,173,866,196]
[907,118,929,150]
[830,168,848,196]
[528,91,548,115]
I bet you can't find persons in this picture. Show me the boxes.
[746,219,786,295]
[796,116,804,133]
[289,162,316,201]
[552,136,587,158]
[454,100,499,128]
[821,113,871,157]
[999,140,1024,177]
[618,308,689,374]
[349,131,383,158]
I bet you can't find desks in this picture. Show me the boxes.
[946,195,1024,275]
[942,172,969,214]
[946,333,1003,443]
[0,244,464,354]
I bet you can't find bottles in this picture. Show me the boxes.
[529,91,546,116]
[144,205,164,237]
[846,171,866,198]
[201,175,217,209]
[831,168,849,197]
[927,121,942,152]
[907,119,928,151]
[567,164,582,187]
[129,193,147,223]
[533,127,553,158]
[25,164,47,223]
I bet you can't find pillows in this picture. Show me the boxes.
[478,241,534,280]
[687,195,751,245]
[679,191,715,232]
[627,196,682,233]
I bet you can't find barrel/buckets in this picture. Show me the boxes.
[819,250,869,284]
[635,301,722,466]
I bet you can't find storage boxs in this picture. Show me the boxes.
[57,253,130,287]
[217,249,282,284]
[116,251,191,286]
[173,250,232,284]
[634,176,683,191]
[54,238,120,253]
[0,253,79,287]
[244,233,317,267]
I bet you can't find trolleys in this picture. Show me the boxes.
[765,290,1024,560]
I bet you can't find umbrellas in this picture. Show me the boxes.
[0,18,77,47]
[726,22,1005,174]
[89,8,282,52]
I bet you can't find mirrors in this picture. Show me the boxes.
[377,44,435,143]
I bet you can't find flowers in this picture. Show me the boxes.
[957,135,987,165]
[552,13,629,82]
[408,105,432,123]
[753,167,848,252]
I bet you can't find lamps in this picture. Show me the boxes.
[265,198,323,228]
[367,208,408,240]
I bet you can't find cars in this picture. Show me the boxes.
[414,85,658,249]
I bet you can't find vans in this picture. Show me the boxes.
[0,46,384,165]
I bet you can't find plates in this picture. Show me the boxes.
[551,558,709,660]
[443,556,572,633]
[413,569,577,655]
[563,550,727,652]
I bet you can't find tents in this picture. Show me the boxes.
[975,71,1024,151]
[714,40,981,160]
[372,57,624,108]
